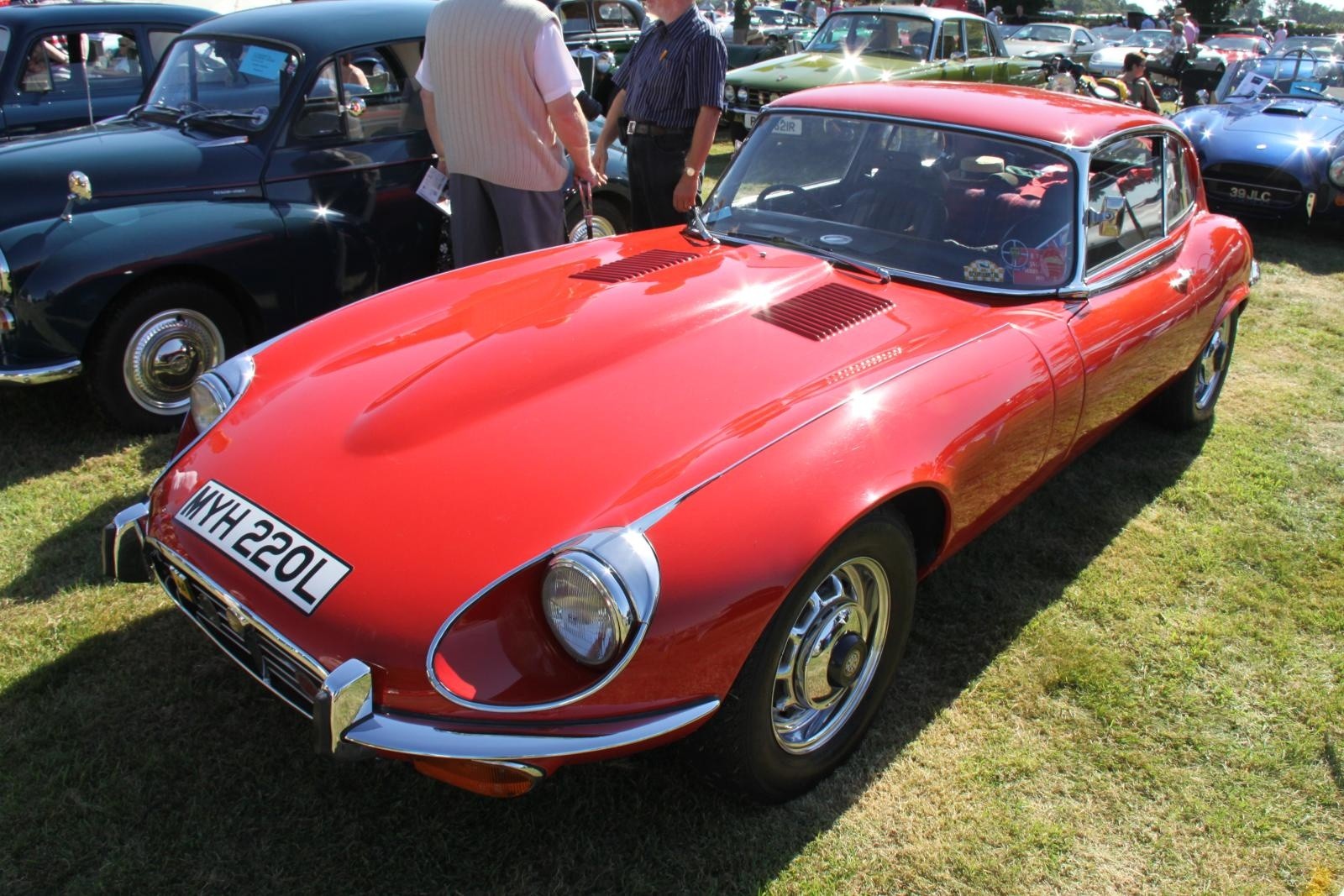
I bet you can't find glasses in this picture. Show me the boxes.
[1139,64,1146,68]
[119,43,130,47]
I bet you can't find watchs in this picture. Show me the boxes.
[685,167,695,176]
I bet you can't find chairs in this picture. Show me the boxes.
[1028,185,1070,255]
[833,183,945,242]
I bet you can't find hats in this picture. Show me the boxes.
[746,30,763,41]
[865,18,887,32]
[1252,18,1258,24]
[1169,21,1186,34]
[992,6,1003,14]
[1171,8,1191,19]
[1278,22,1286,29]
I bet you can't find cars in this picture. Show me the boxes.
[1089,29,1177,77]
[1197,34,1273,65]
[1244,37,1344,87]
[1167,55,1343,220]
[787,15,879,54]
[0,3,375,142]
[1327,34,1344,45]
[540,0,655,50]
[720,5,810,46]
[1,1,629,431]
[1088,26,1137,48]
[997,22,1104,72]
[723,4,1049,141]
[98,77,1257,799]
[1187,43,1226,72]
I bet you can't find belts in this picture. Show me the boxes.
[627,120,695,136]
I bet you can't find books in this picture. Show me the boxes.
[416,165,452,216]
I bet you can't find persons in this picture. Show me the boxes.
[108,37,135,75]
[1117,52,1161,113]
[1116,17,1124,26]
[1252,18,1263,36]
[1171,8,1200,46]
[1274,21,1288,45]
[414,0,596,269]
[701,0,734,11]
[1011,5,1028,25]
[1142,13,1167,29]
[1166,20,1188,66]
[781,0,856,26]
[323,52,372,95]
[987,6,1004,22]
[592,0,728,232]
[34,0,89,74]
[732,0,757,41]
